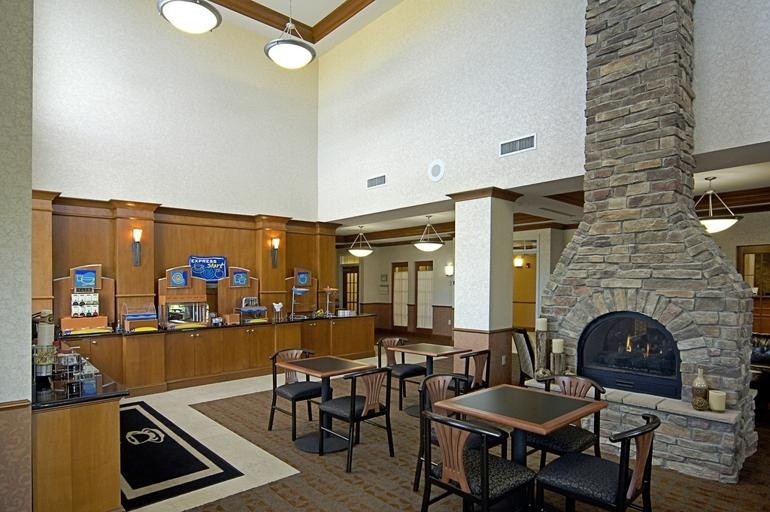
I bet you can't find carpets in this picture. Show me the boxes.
[119,401,244,512]
[183,357,585,512]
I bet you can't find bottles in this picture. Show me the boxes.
[692,368,708,409]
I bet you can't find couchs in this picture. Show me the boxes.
[750,333,770,372]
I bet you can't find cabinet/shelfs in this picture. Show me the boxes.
[61,336,123,394]
[165,328,223,391]
[301,320,330,356]
[330,316,376,360]
[223,324,275,381]
[32,396,125,512]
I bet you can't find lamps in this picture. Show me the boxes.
[264,0,317,70]
[271,237,280,268]
[694,176,744,235]
[346,226,374,257]
[444,263,454,276]
[411,215,446,252]
[513,256,523,268]
[132,229,143,266]
[157,0,221,34]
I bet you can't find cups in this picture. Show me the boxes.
[709,390,725,411]
[212,318,219,328]
